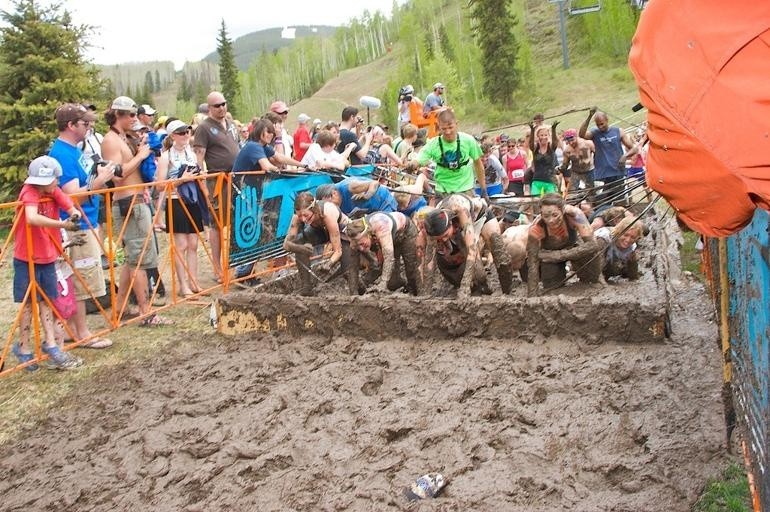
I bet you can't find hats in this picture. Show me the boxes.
[500,133,509,142]
[562,129,577,140]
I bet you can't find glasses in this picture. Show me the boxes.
[508,145,515,148]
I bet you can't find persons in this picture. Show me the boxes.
[625,1,769,239]
[14,156,82,374]
[416,83,653,297]
[46,85,422,348]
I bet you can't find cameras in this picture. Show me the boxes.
[357,117,363,123]
[177,164,202,179]
[398,88,412,103]
[449,161,458,169]
[90,153,124,179]
[367,126,374,132]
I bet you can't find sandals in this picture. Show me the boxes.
[12,276,224,371]
